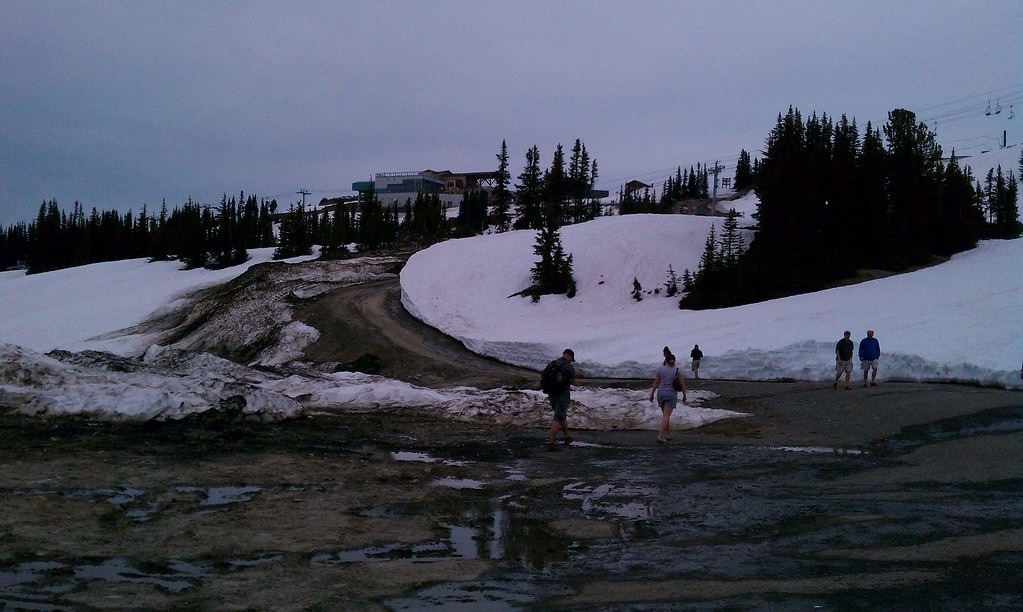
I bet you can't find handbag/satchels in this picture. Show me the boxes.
[672,368,682,392]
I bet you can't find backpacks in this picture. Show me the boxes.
[540,360,571,395]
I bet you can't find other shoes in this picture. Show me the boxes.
[545,443,560,451]
[665,434,675,440]
[564,437,574,443]
[657,434,667,442]
[863,384,867,388]
[870,383,879,388]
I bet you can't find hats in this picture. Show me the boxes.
[844,331,851,336]
[563,349,575,362]
[666,354,676,362]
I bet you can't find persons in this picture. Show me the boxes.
[649,346,687,442]
[858,330,881,388]
[690,344,703,379]
[542,349,579,450]
[833,331,856,389]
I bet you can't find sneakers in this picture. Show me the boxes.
[831,381,837,390]
[844,384,852,390]
